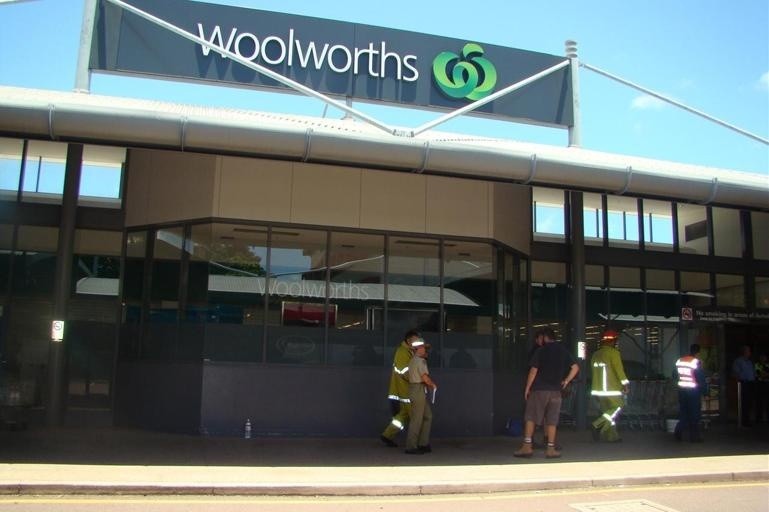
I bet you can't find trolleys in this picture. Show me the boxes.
[560,376,712,431]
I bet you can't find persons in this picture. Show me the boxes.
[407,340,439,455]
[587,328,631,443]
[528,331,564,450]
[674,343,710,441]
[380,330,419,447]
[733,345,757,428]
[520,328,580,459]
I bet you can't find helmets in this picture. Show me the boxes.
[602,329,619,340]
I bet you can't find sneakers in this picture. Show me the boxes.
[406,446,431,454]
[545,448,561,458]
[513,447,532,457]
[592,424,601,440]
[380,434,397,447]
